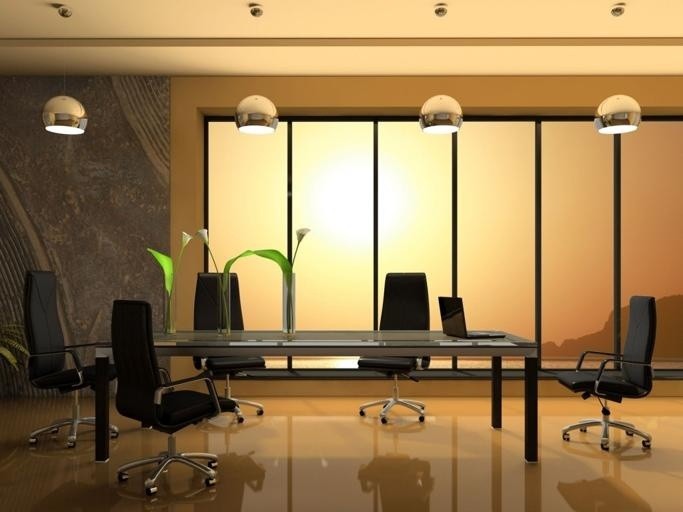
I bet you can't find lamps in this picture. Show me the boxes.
[419,8,464,135]
[594,6,642,136]
[42,6,88,135]
[234,7,279,134]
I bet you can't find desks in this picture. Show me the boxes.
[95,330,539,464]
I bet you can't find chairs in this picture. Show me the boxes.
[111,300,236,496]
[559,295,657,452]
[22,270,119,450]
[193,273,265,424]
[557,432,652,512]
[358,416,436,512]
[194,415,265,512]
[357,272,430,424]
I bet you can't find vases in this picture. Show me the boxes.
[282,272,296,338]
[216,273,231,337]
[162,279,177,335]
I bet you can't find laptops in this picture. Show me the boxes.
[437,295,507,340]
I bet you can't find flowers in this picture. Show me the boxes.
[253,228,312,333]
[195,228,256,332]
[146,231,192,328]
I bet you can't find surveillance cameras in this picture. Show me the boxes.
[434,4,449,17]
[250,4,264,17]
[57,6,73,18]
[610,5,626,17]
